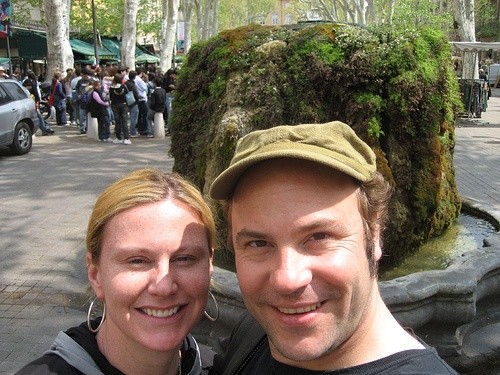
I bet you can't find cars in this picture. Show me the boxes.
[0,78,39,156]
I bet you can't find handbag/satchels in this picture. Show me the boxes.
[125,91,136,106]
[50,95,55,106]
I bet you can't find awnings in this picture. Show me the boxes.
[35,33,160,64]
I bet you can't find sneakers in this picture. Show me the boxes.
[124,139,131,144]
[139,132,154,138]
[112,138,123,144]
[98,138,112,141]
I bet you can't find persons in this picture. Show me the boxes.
[209,120,460,375]
[15,170,224,375]
[1,64,180,145]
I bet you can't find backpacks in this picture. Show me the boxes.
[80,87,94,110]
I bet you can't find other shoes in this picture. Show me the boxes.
[61,120,70,126]
[130,134,140,137]
[42,128,55,135]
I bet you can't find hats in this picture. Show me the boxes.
[209,120,377,201]
[119,65,129,70]
[0,65,4,71]
